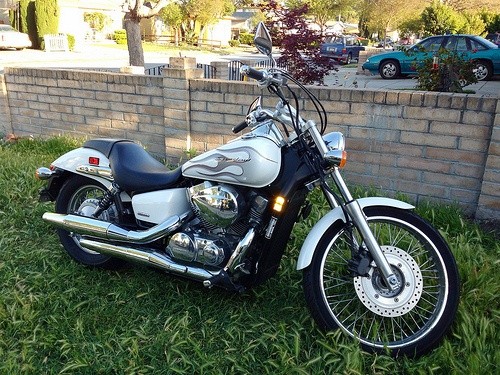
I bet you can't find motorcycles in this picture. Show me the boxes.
[35,21,460,366]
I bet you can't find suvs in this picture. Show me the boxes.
[316,34,365,66]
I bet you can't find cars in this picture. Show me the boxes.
[377,37,394,47]
[0,24,32,51]
[360,32,500,82]
[398,37,412,45]
